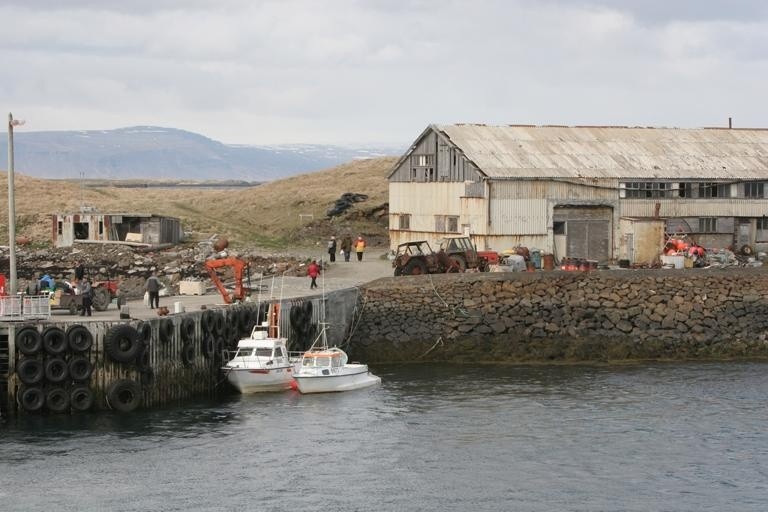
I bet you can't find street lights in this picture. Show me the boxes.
[7,112,27,311]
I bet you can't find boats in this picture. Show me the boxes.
[221,272,304,395]
[294,256,382,395]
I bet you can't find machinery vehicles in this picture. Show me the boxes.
[26,265,118,315]
[438,235,498,273]
[392,241,464,277]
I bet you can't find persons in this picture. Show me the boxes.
[341,233,353,262]
[328,235,337,263]
[25,260,83,298]
[306,257,321,289]
[81,276,92,316]
[355,235,367,261]
[145,273,162,308]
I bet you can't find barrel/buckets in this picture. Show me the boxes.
[526,251,599,272]
